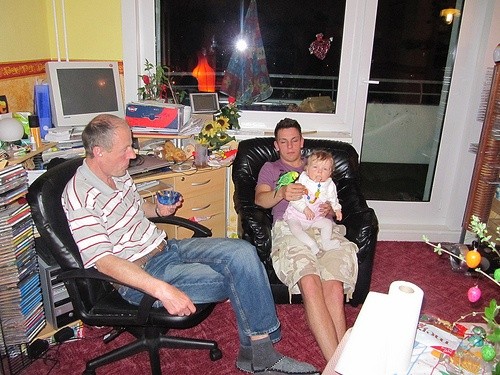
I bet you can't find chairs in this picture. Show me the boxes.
[26,157,222,375]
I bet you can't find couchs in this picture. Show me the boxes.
[231,137,379,308]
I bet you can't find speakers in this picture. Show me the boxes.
[189,93,220,114]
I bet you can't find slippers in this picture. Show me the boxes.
[236,356,319,375]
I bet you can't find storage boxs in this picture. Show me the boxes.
[126,102,191,133]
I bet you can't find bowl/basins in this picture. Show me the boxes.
[172,160,193,172]
[156,190,181,205]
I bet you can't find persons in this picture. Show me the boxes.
[61,114,318,375]
[254,118,359,361]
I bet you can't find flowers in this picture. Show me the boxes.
[193,102,244,153]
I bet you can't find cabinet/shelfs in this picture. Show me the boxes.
[0,135,238,344]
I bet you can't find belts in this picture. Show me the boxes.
[133,239,167,267]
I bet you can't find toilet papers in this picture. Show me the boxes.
[335,280,425,375]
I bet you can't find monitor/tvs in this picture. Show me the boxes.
[44,61,125,130]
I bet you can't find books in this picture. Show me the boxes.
[0,164,47,344]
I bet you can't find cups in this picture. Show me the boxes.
[195,144,208,168]
